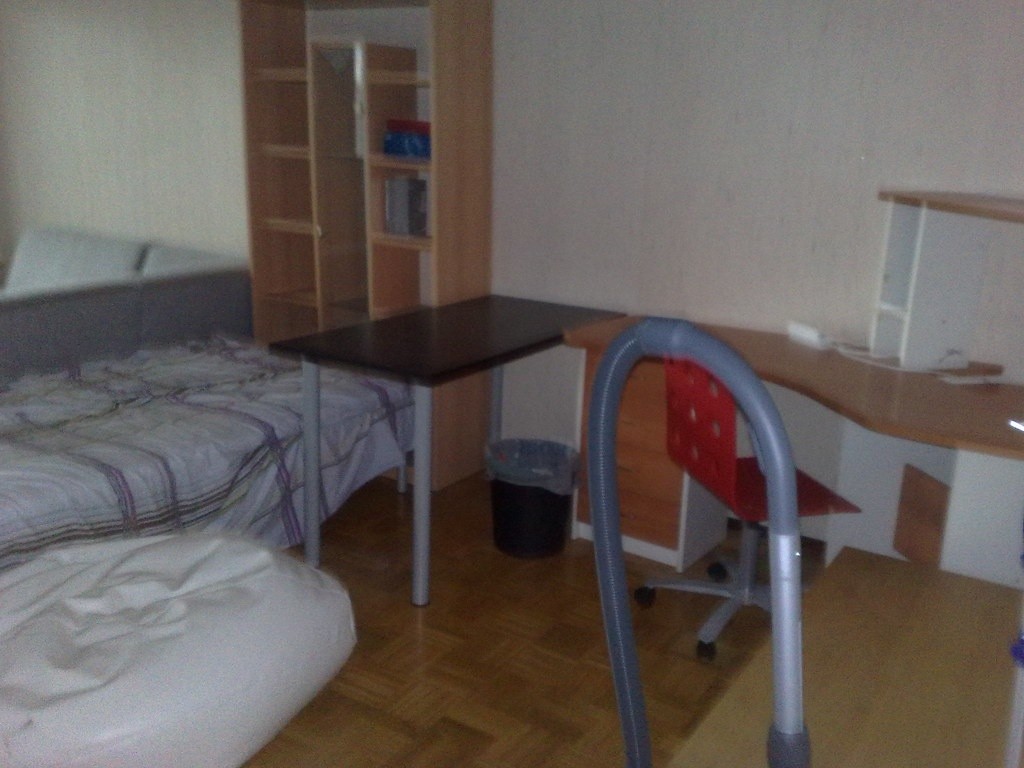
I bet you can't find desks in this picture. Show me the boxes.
[266,294,628,606]
[563,313,1024,572]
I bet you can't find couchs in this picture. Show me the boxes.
[1,228,253,391]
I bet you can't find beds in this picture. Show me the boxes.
[0,532,359,768]
[1,329,415,555]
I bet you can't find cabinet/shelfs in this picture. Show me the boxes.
[240,0,489,490]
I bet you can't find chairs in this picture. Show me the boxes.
[634,356,862,660]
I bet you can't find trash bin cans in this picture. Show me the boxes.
[482,437,577,555]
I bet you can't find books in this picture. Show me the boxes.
[384,176,428,237]
[384,120,431,165]
